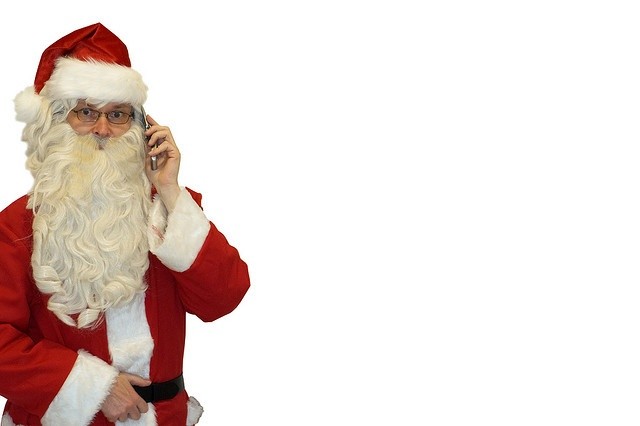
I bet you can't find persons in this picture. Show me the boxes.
[2,21,249,426]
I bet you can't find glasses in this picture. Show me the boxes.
[70,107,135,125]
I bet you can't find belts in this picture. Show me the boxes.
[132,373,184,403]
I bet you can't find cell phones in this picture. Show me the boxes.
[138,104,161,171]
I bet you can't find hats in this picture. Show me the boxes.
[13,23,149,120]
